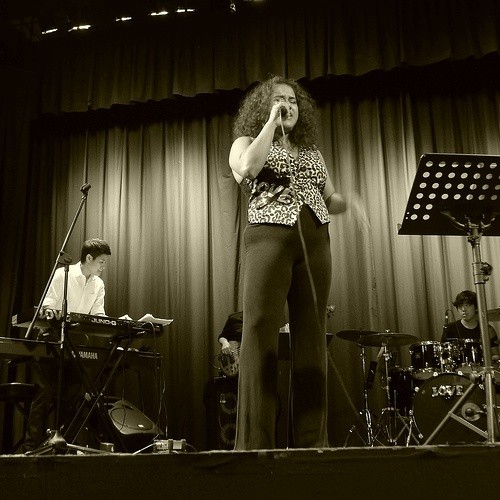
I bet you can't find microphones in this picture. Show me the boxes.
[279,106,288,116]
[443,309,450,329]
[465,407,485,417]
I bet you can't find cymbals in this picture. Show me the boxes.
[358,331,421,347]
[336,329,380,341]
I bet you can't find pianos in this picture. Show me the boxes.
[0,312,166,458]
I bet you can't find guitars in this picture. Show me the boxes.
[219,304,336,380]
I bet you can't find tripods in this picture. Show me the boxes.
[24,182,109,456]
[344,153,500,449]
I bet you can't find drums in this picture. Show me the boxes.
[408,340,444,375]
[408,370,490,444]
[452,339,487,371]
[388,366,426,421]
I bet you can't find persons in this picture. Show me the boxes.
[227,76,368,450]
[218,311,244,356]
[22,239,111,455]
[441,290,500,361]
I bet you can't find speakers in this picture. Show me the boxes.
[100,395,165,453]
[203,377,237,451]
